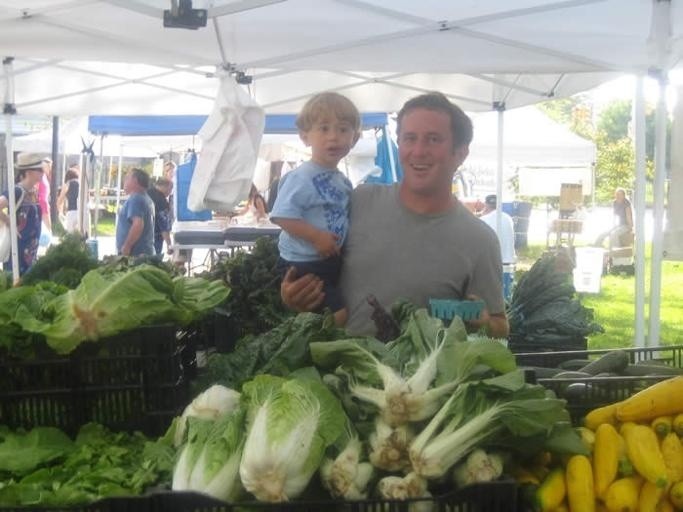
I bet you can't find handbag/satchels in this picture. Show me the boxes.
[1,219,11,264]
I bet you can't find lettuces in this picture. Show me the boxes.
[1,263,232,362]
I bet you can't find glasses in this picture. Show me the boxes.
[25,168,42,171]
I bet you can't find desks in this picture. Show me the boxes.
[169,239,257,277]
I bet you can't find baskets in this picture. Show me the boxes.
[511,344,682,429]
[0,479,546,511]
[1,285,235,441]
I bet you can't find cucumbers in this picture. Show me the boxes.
[534,350,683,401]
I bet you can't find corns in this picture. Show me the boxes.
[536,375,683,512]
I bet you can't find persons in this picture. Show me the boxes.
[477,192,516,298]
[35,156,52,253]
[54,168,81,237]
[145,174,176,258]
[113,166,157,259]
[0,150,49,281]
[264,91,362,330]
[280,91,513,348]
[612,187,635,235]
[70,163,92,240]
[162,160,175,177]
[230,182,267,221]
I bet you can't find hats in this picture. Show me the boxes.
[13,149,49,171]
[485,195,496,204]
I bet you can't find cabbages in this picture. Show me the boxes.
[174,305,589,511]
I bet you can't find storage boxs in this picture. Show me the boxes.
[572,247,604,293]
